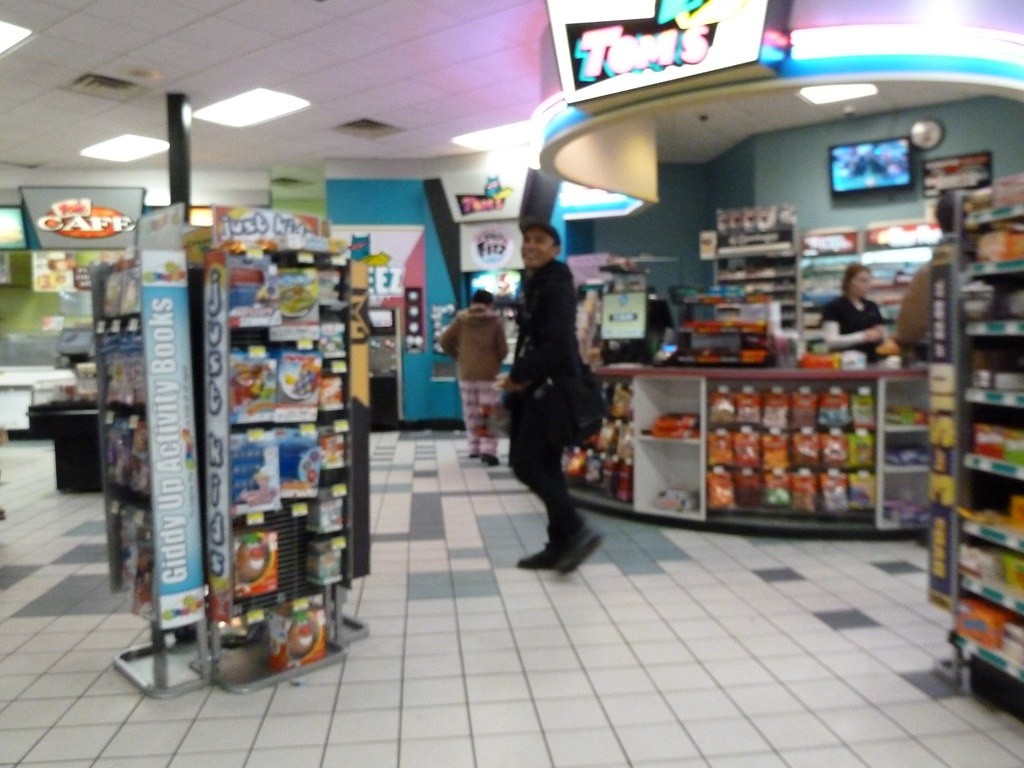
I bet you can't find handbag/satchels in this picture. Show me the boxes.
[549,365,607,446]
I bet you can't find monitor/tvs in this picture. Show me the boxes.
[828,137,916,198]
[0,206,29,251]
[648,300,673,334]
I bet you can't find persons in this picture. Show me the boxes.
[821,263,886,352]
[439,290,508,466]
[501,218,603,575]
[897,195,954,343]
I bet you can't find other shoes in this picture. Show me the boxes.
[547,515,601,574]
[481,454,498,465]
[517,543,552,569]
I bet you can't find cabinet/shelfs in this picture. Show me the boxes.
[926,183,1024,709]
[714,226,942,351]
[90,211,370,701]
